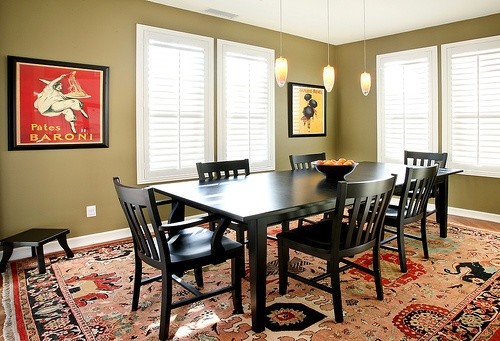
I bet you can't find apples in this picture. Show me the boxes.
[314,158,354,165]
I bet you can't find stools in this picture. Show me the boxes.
[0,229,74,273]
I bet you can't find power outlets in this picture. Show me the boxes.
[87,205,96,218]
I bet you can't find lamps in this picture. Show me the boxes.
[360,0,371,97]
[275,0,288,88]
[323,0,335,92]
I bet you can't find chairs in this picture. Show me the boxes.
[113,151,448,340]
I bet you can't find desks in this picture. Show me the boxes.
[148,162,463,332]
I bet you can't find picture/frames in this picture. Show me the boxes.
[288,82,326,137]
[7,55,109,151]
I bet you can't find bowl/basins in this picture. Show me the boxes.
[313,164,359,184]
